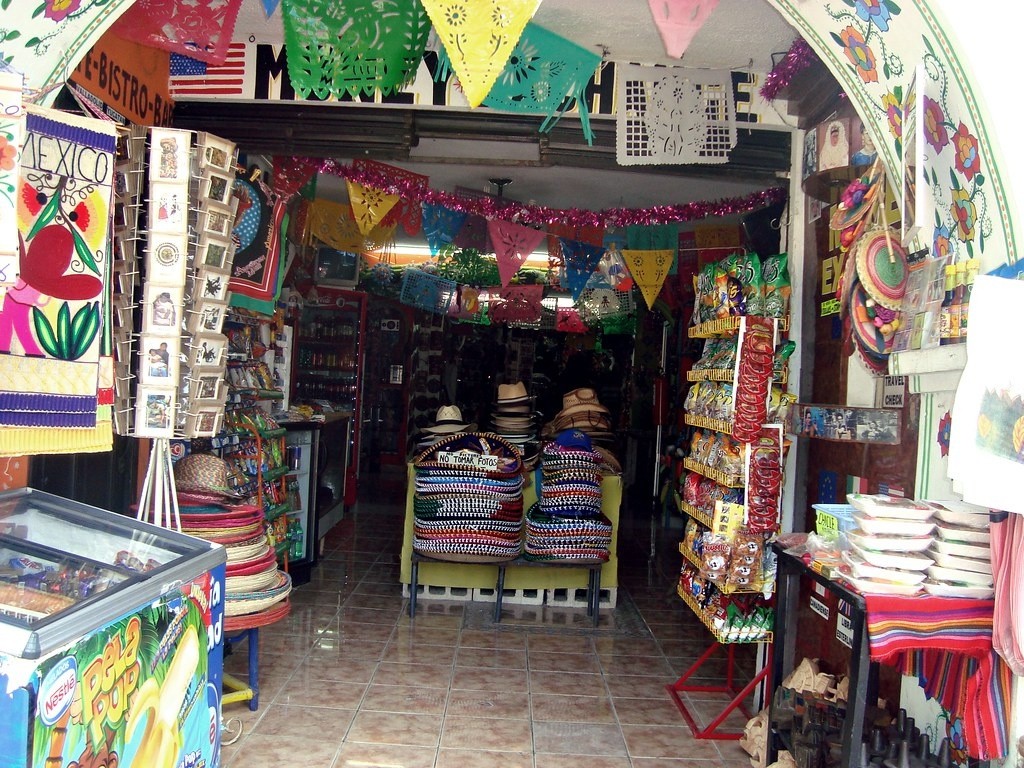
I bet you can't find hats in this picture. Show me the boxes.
[553,388,611,435]
[524,441,612,564]
[491,419,536,428]
[168,454,243,499]
[130,492,292,631]
[412,432,524,562]
[556,429,593,452]
[496,427,541,471]
[592,445,621,473]
[421,405,471,434]
[491,381,540,429]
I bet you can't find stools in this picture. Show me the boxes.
[222,599,292,714]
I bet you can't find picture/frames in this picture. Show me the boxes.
[196,198,235,237]
[112,130,134,434]
[190,366,226,402]
[188,299,228,333]
[187,332,229,368]
[192,234,232,270]
[187,400,226,437]
[134,129,190,438]
[196,164,235,205]
[192,265,231,301]
[197,131,237,173]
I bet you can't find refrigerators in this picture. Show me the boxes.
[367,295,416,462]
[290,282,368,509]
[0,487,228,768]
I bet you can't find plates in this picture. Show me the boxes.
[836,494,994,597]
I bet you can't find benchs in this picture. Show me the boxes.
[411,552,602,626]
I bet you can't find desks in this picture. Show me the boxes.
[767,543,994,768]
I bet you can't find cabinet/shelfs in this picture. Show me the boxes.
[113,125,239,533]
[668,316,792,739]
[171,295,355,589]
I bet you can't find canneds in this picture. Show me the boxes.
[286,445,301,470]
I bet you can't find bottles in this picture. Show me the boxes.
[297,314,356,400]
[369,391,401,453]
